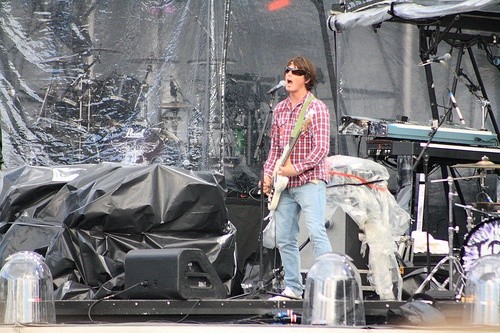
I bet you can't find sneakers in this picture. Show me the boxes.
[268,287,302,301]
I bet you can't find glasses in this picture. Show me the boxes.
[284,66,307,76]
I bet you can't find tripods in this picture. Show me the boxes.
[408,170,487,303]
[227,93,303,300]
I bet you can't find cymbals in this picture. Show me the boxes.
[465,199,500,211]
[450,159,500,169]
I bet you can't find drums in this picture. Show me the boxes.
[459,215,500,281]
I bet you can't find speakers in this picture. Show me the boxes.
[124,248,228,300]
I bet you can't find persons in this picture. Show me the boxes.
[262,55,333,300]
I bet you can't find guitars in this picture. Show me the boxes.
[267,110,314,212]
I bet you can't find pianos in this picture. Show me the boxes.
[337,114,498,147]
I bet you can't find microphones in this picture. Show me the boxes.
[266,80,286,95]
[324,219,334,230]
[485,194,493,202]
[424,53,451,63]
[449,90,465,124]
